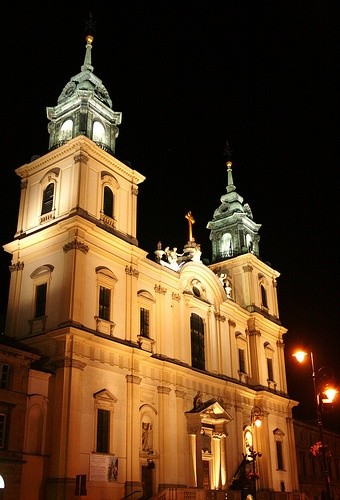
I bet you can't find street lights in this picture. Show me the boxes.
[249,405,264,500]
[291,339,339,500]
